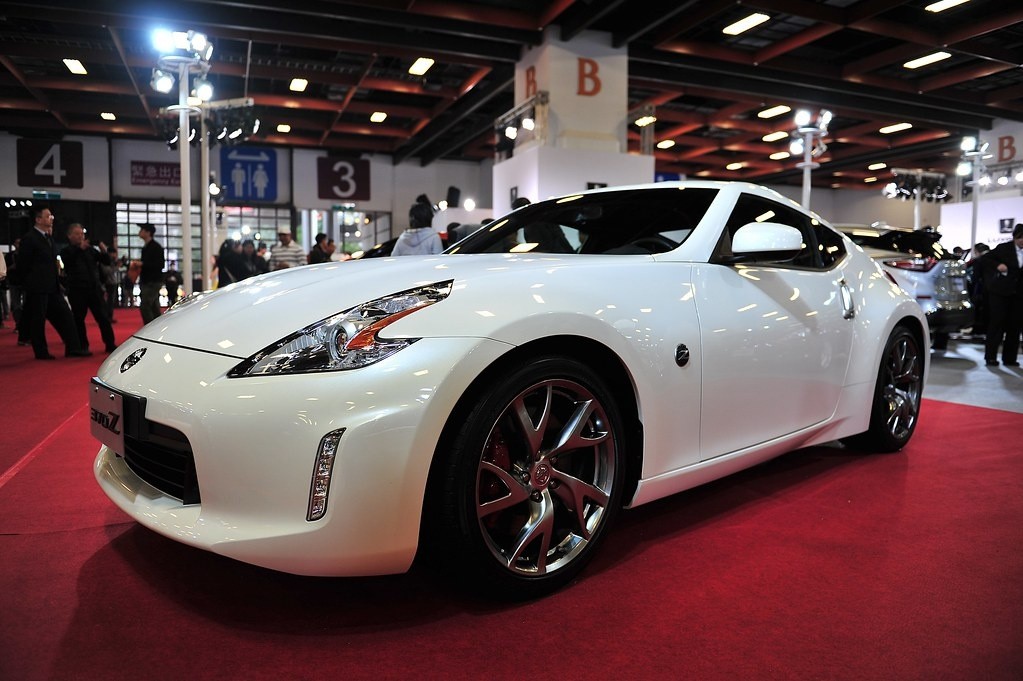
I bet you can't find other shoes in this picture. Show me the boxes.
[34,354,55,360]
[109,320,117,323]
[1003,362,1019,366]
[17,340,24,345]
[64,350,92,357]
[986,359,999,365]
[106,345,117,353]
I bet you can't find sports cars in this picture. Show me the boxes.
[88,178,933,598]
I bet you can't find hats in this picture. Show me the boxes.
[277,225,290,235]
[136,223,156,233]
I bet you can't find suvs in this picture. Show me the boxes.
[839,225,972,350]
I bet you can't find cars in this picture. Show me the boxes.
[342,231,449,256]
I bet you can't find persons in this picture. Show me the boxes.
[963,224,1023,367]
[100,248,142,323]
[511,198,574,253]
[17,207,92,360]
[0,234,21,334]
[215,239,274,288]
[953,246,963,256]
[60,223,118,356]
[164,261,184,305]
[136,223,164,325]
[309,232,335,265]
[269,224,308,272]
[391,204,444,254]
